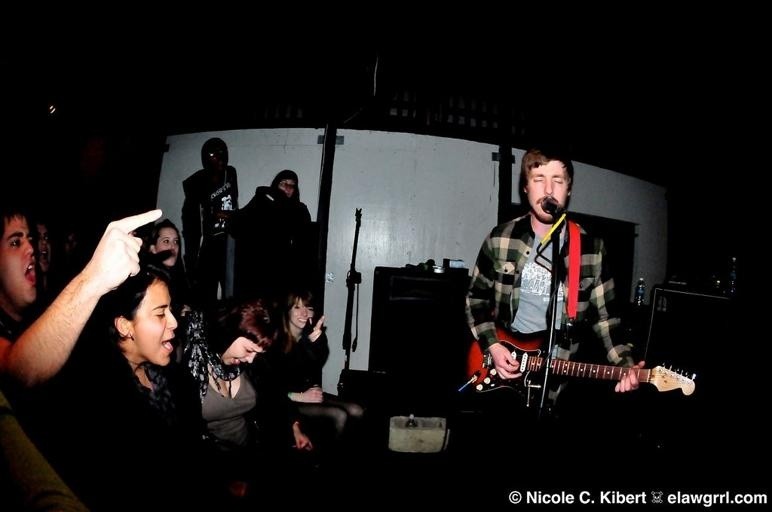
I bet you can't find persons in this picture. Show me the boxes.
[0,136,364,512]
[464,144,646,501]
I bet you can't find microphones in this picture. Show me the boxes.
[540,196,562,214]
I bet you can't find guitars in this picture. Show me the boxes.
[468,327,698,399]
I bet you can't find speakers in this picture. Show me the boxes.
[642,283,730,382]
[368,264,470,386]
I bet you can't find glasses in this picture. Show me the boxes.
[279,182,295,189]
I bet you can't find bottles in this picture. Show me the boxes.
[726,257,739,297]
[405,414,418,427]
[634,276,646,306]
